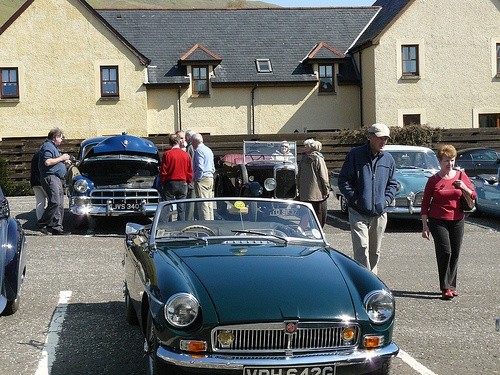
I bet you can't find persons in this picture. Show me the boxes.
[191,133,216,221]
[298,140,331,228]
[269,140,299,176]
[185,129,197,221]
[420,145,478,298]
[35,127,72,235]
[30,139,50,221]
[413,152,427,169]
[391,152,404,168]
[158,133,194,223]
[174,129,189,153]
[337,123,398,276]
[303,137,328,229]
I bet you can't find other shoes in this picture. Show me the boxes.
[442,289,458,298]
[52,230,71,235]
[36,222,49,235]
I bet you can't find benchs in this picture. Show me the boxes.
[222,153,272,164]
[144,220,297,237]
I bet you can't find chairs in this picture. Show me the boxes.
[233,228,288,237]
[163,229,210,237]
[234,158,254,165]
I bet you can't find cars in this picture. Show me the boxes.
[214,139,300,224]
[1,186,28,317]
[454,146,500,218]
[328,144,442,226]
[64,131,161,234]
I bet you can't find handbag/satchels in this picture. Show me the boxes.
[459,172,476,212]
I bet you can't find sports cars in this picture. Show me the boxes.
[121,197,401,375]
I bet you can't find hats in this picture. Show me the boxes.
[368,124,391,139]
